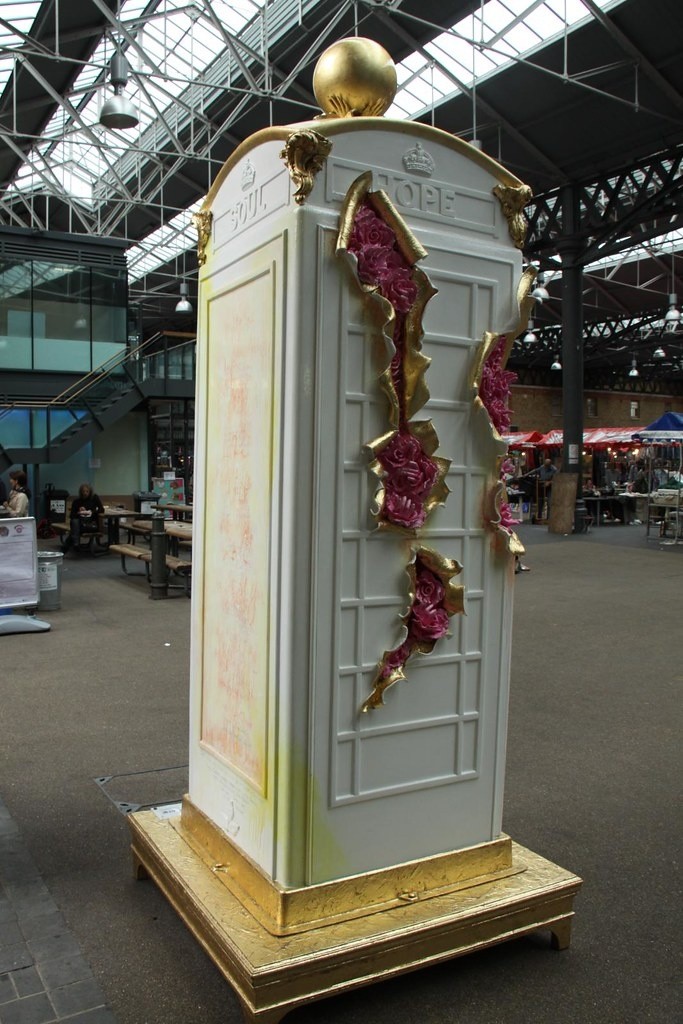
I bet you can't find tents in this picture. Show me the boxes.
[500,410,683,546]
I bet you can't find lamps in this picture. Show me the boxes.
[100,51,140,128]
[665,294,681,321]
[550,354,562,370]
[653,348,665,357]
[523,320,538,343]
[628,359,639,376]
[176,283,193,315]
[532,273,550,299]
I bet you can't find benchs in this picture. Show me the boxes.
[51,518,192,598]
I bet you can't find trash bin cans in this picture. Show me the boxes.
[133,491,162,520]
[36,551,65,612]
[43,489,70,522]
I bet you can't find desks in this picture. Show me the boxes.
[98,505,142,549]
[585,494,659,526]
[132,520,192,590]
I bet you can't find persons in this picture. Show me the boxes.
[60,484,105,558]
[631,459,649,525]
[522,459,559,520]
[3,470,29,518]
[583,476,617,520]
